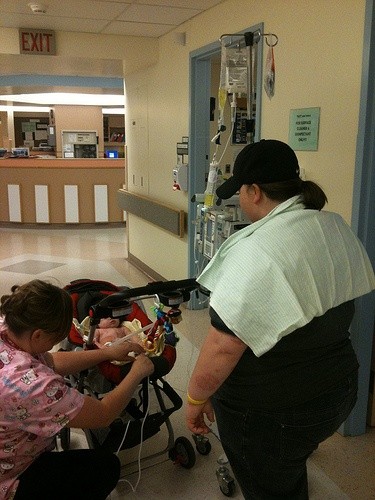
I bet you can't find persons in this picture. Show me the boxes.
[84,296,142,350]
[0,279,155,500]
[186,138,360,498]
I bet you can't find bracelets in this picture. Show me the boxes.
[186,388,209,404]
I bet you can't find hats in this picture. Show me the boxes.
[216,139,299,200]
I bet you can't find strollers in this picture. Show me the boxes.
[62,279,200,479]
